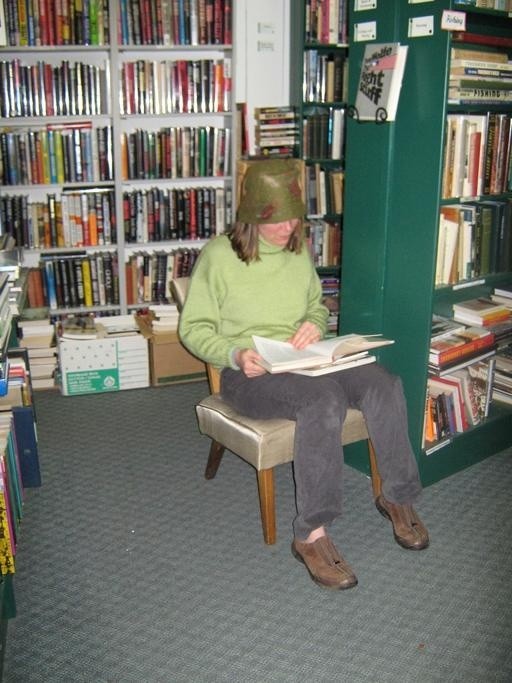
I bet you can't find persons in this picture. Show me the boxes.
[177,156,430,589]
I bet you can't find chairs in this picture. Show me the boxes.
[168,273,382,546]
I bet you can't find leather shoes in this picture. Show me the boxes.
[376,495,430,550]
[292,536,358,590]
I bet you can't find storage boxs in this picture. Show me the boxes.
[136,314,208,387]
[56,321,150,396]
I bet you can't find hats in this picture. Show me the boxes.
[236,161,307,224]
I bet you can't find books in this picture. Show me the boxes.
[117,0,231,329]
[303,0,349,327]
[422,287,512,454]
[453,32,512,48]
[459,0,512,12]
[1,1,119,317]
[255,104,295,156]
[434,48,511,285]
[0,271,143,576]
[353,42,409,120]
[247,332,396,379]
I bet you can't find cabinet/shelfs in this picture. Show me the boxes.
[333,0,512,487]
[0,0,116,320]
[291,0,347,279]
[116,0,235,317]
